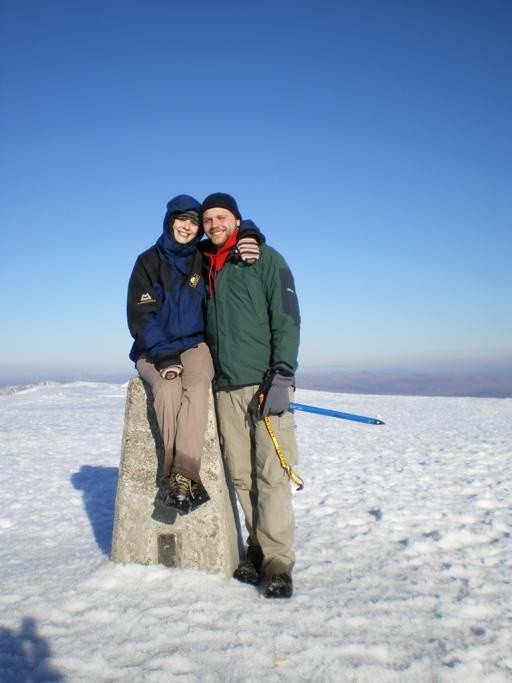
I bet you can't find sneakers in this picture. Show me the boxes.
[234,548,262,585]
[266,573,293,597]
[160,474,210,513]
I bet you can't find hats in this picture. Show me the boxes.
[203,193,243,221]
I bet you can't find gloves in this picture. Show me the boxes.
[234,236,261,264]
[160,364,182,379]
[258,369,293,417]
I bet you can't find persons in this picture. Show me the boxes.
[201,194,301,599]
[124,194,266,516]
